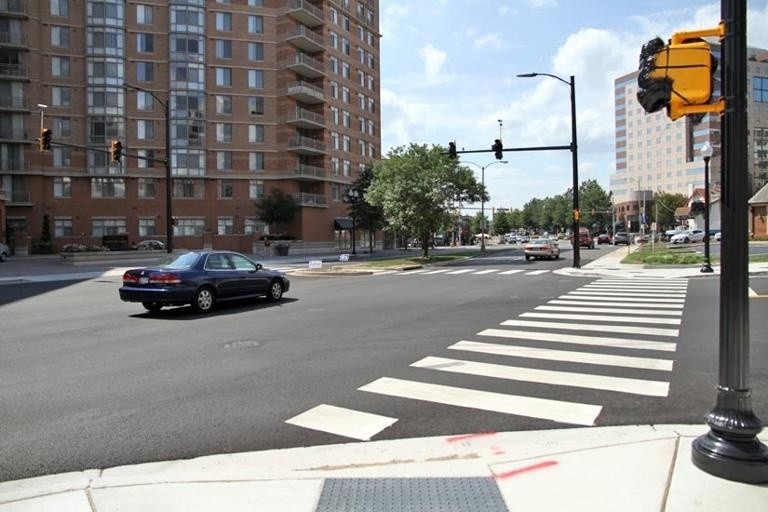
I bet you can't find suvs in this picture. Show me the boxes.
[571,228,595,249]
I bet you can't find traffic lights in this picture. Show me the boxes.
[448,142,456,159]
[495,139,503,159]
[112,140,122,163]
[41,128,51,153]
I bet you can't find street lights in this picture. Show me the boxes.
[504,232,530,243]
[482,160,508,244]
[516,73,580,265]
[348,189,358,254]
[624,177,643,239]
[701,142,714,267]
[122,82,172,251]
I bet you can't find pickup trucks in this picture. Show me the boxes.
[666,226,687,235]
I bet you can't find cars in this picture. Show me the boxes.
[670,229,706,244]
[117,250,289,315]
[0,241,11,262]
[598,234,611,245]
[614,233,632,245]
[130,240,165,250]
[523,236,560,261]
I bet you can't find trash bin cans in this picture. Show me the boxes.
[278,243,289,256]
